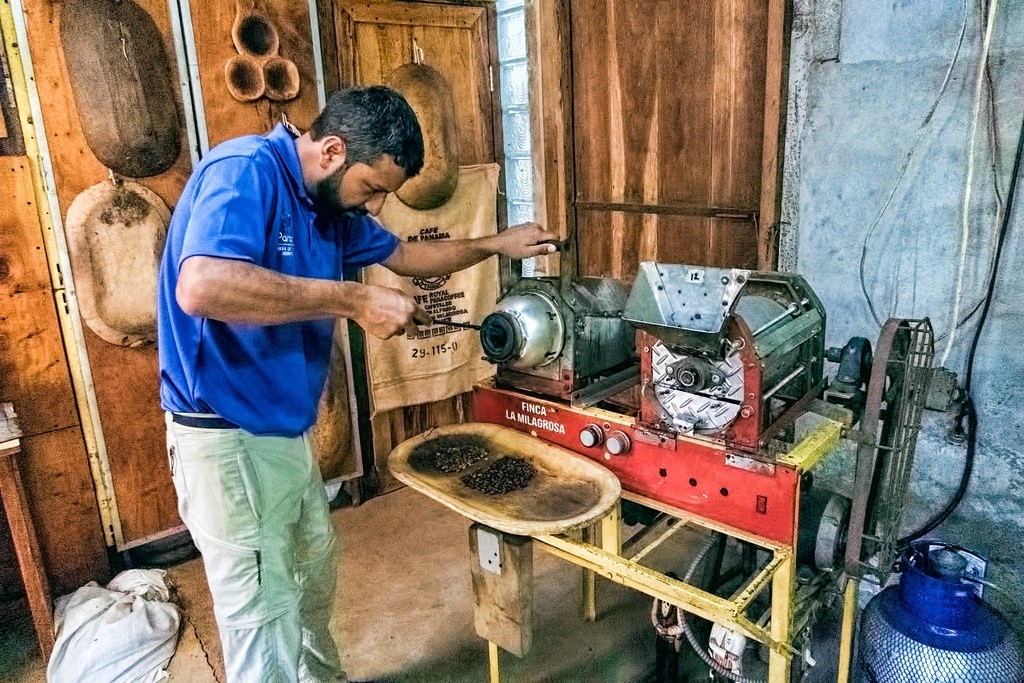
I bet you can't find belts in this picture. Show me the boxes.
[171,412,240,429]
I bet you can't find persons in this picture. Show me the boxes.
[157,90,555,683]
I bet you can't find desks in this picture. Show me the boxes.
[0,438,57,665]
